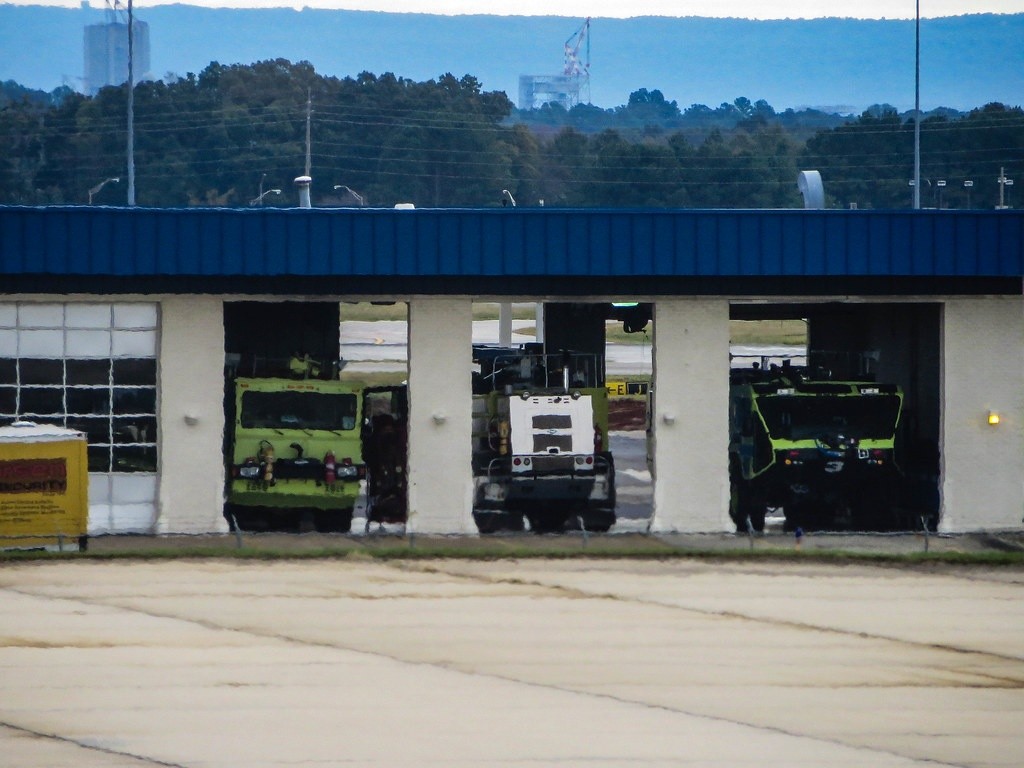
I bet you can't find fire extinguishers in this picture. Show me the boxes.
[324,449,335,484]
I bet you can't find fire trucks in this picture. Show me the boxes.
[226,352,361,534]
[729,351,906,532]
[363,341,620,538]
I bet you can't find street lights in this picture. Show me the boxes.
[88,177,120,205]
[250,189,282,207]
[334,184,364,207]
